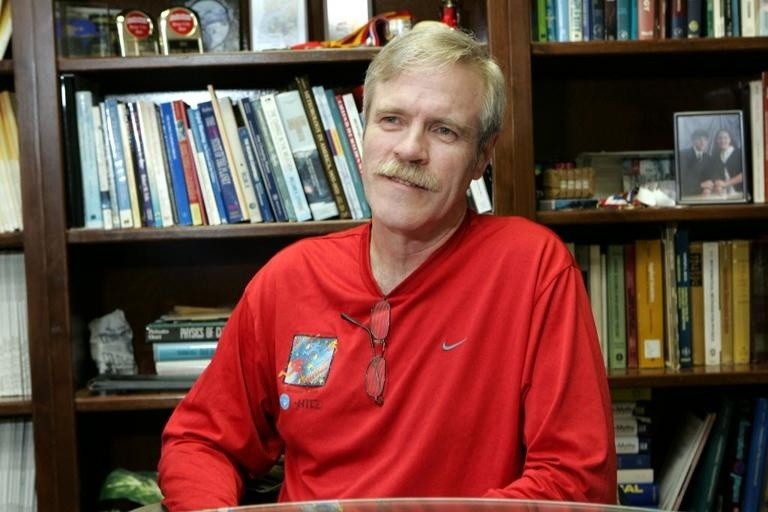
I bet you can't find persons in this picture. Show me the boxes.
[711,130,743,197]
[679,130,713,197]
[158,20,617,512]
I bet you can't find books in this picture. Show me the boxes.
[750,81,765,204]
[87,306,237,393]
[75,75,372,230]
[535,1,768,43]
[612,383,768,511]
[564,238,768,370]
[0,1,38,512]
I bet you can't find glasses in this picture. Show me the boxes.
[340,297,391,407]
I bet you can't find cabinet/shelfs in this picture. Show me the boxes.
[0,0,767,512]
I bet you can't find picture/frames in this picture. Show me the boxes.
[672,109,748,205]
